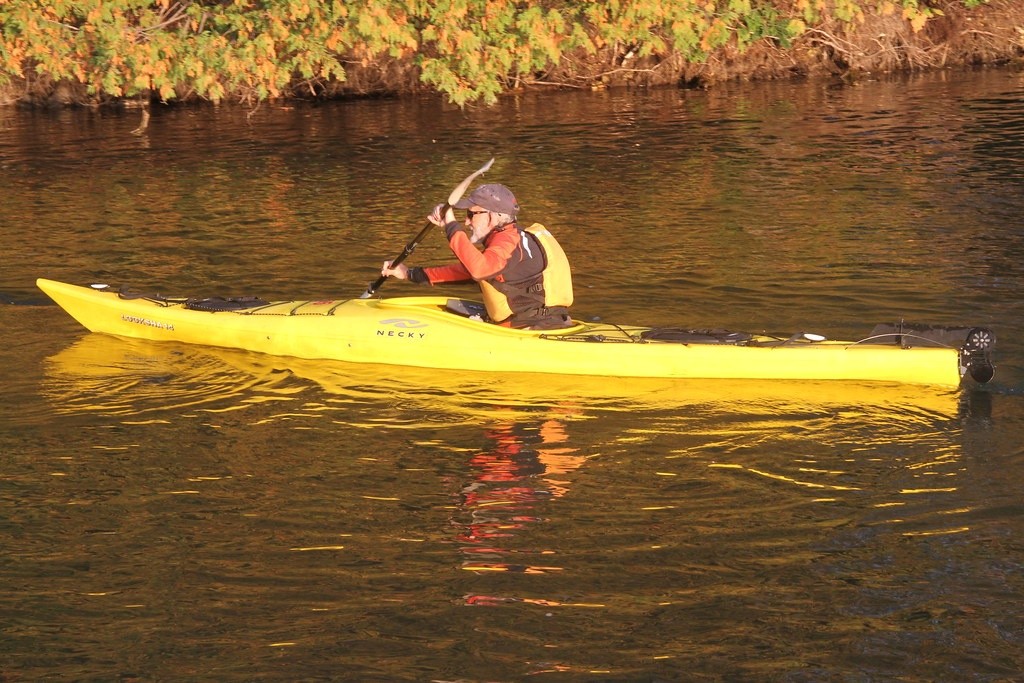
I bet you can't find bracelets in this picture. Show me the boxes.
[408,268,413,281]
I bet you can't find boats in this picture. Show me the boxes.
[35,278,998,389]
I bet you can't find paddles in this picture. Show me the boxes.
[358,157,499,298]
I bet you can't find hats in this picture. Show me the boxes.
[454,184,520,216]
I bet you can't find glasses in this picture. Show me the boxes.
[467,209,501,218]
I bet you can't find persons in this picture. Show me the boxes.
[381,184,548,328]
